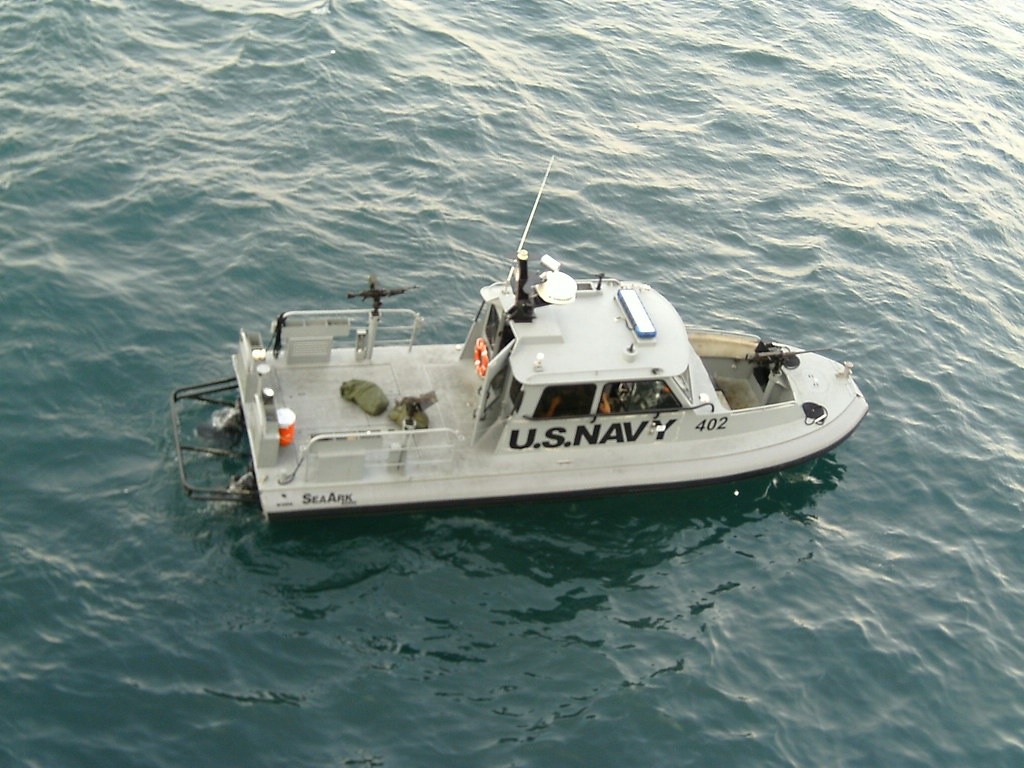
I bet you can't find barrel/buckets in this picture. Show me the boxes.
[275,407,296,446]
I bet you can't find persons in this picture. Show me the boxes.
[598,391,621,414]
[536,391,575,417]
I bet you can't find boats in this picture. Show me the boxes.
[169,155,870,525]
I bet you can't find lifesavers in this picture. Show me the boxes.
[474,338,489,376]
